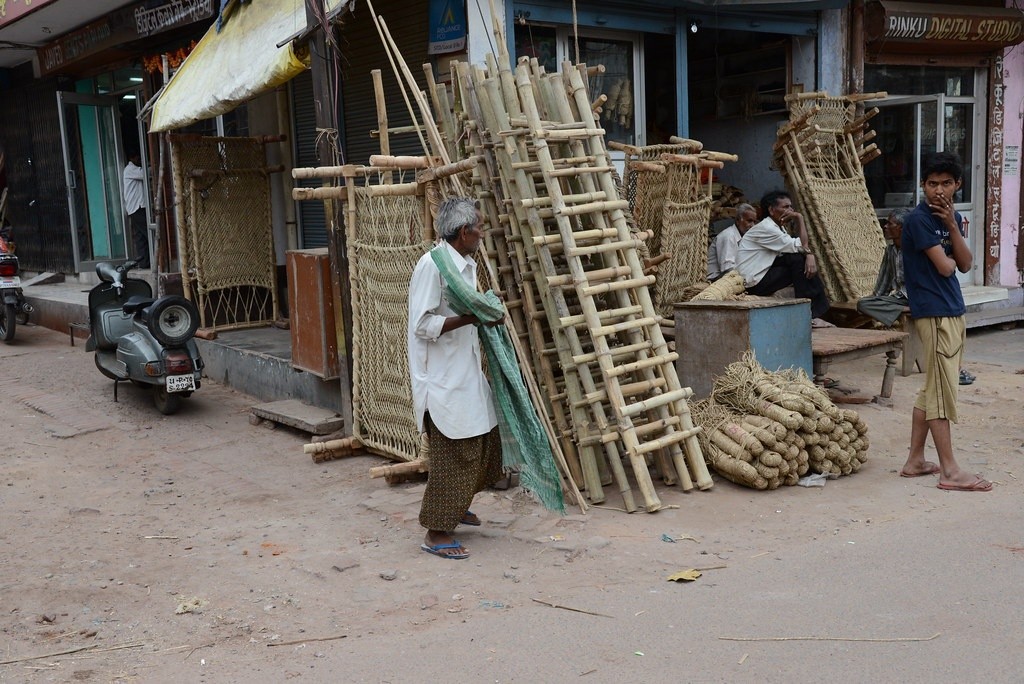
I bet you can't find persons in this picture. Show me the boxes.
[707,203,756,283]
[857,207,909,327]
[899,151,994,491]
[735,193,838,327]
[408,196,507,560]
[123,149,150,269]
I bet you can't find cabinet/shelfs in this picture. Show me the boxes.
[688,42,795,126]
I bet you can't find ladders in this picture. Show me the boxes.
[445,56,713,514]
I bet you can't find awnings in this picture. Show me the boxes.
[148,0,355,132]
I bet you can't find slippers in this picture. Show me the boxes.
[959,368,976,384]
[812,375,841,388]
[899,460,940,477]
[937,471,993,491]
[459,510,481,526]
[421,537,470,559]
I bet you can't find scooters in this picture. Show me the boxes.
[85,256,208,415]
[0,226,34,340]
